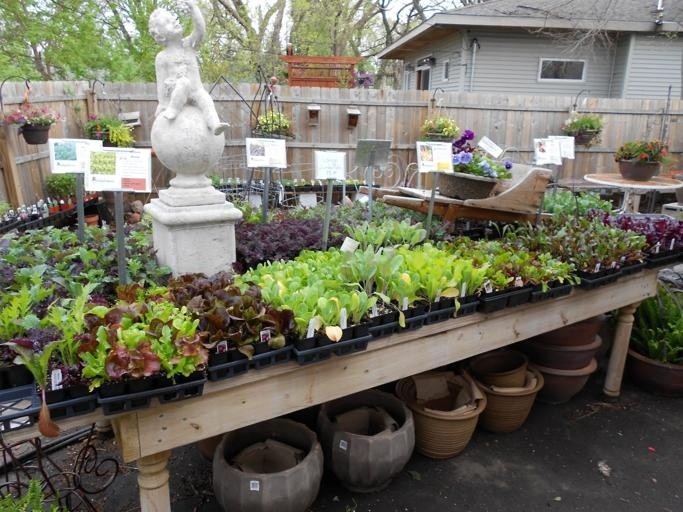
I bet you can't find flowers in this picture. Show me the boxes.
[449,128,515,180]
[614,138,675,166]
[0,101,61,128]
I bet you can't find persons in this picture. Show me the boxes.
[147,0,230,136]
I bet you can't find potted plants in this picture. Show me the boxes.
[252,111,291,153]
[561,113,605,149]
[611,281,682,396]
[80,113,136,155]
[420,114,459,143]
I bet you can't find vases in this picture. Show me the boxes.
[474,349,531,389]
[528,360,598,407]
[84,215,98,226]
[616,158,660,182]
[541,315,600,344]
[467,362,544,436]
[524,333,602,369]
[396,370,488,463]
[214,419,322,512]
[319,388,417,496]
[438,172,495,199]
[22,123,50,145]
